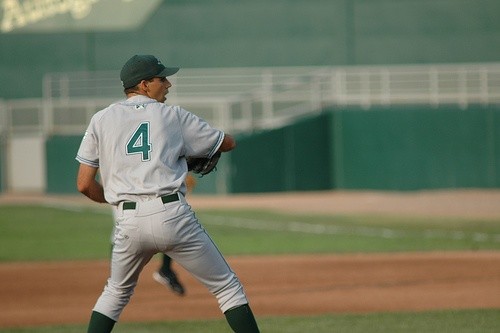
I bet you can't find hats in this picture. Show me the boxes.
[120,55,180,88]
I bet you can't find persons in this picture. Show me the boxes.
[152,175,192,296]
[75,55,260,333]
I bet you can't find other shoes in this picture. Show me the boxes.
[153,269,184,297]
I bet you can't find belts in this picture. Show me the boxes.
[123,193,180,211]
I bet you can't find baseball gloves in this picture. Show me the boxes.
[187,149,222,175]
